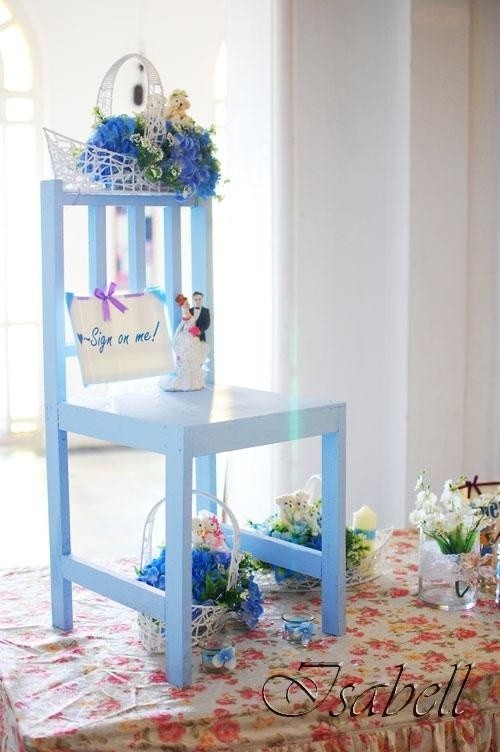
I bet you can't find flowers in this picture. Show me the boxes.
[293,622,316,648]
[210,646,237,671]
[250,501,378,581]
[409,472,499,552]
[75,108,225,203]
[140,538,265,638]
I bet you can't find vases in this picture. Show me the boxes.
[418,540,481,610]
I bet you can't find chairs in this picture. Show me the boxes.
[34,179,348,689]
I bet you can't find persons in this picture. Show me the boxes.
[158,291,210,391]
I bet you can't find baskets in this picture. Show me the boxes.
[43,53,183,192]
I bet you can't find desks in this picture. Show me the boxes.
[1,530,500,751]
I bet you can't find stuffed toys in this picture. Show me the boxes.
[193,510,225,549]
[147,89,190,123]
[274,491,320,536]
